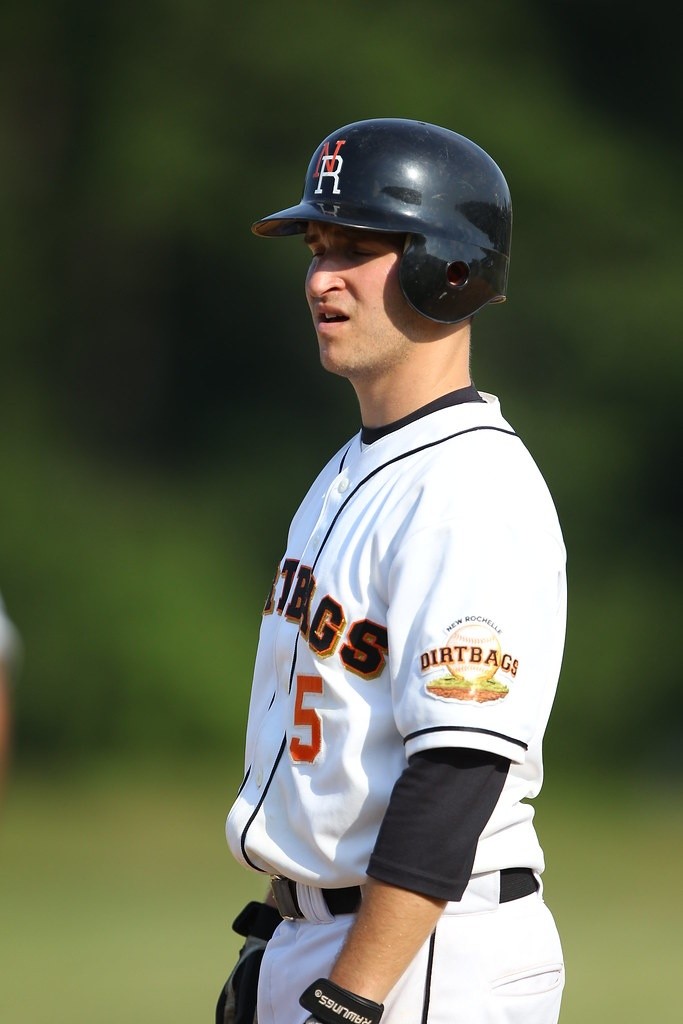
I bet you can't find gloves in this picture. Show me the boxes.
[298,977,385,1024]
[215,900,285,1024]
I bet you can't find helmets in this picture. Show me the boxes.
[249,116,515,326]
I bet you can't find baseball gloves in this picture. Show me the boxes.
[214,901,282,1024]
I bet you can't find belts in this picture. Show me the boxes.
[270,866,540,920]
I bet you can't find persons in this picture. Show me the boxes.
[216,117,565,1023]
